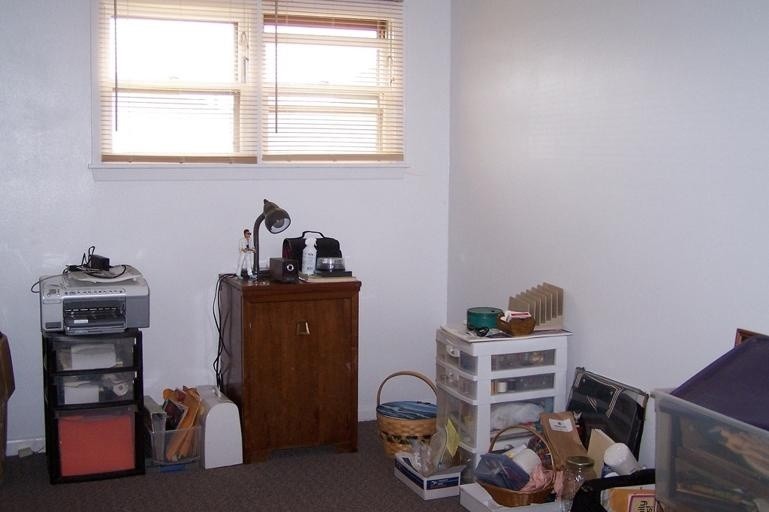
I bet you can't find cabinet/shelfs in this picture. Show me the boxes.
[40,327,146,487]
[216,274,362,465]
[434,324,573,470]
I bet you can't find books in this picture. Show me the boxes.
[159,385,203,465]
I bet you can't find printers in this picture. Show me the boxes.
[38,264,151,336]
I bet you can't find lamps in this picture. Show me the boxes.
[252,199,292,275]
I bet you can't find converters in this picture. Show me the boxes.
[87,253,110,271]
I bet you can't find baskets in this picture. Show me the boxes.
[474,425,556,507]
[496,313,536,336]
[376,371,440,458]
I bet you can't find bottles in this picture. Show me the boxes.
[559,455,599,512]
[301,236,346,275]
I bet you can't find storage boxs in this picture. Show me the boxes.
[145,422,202,474]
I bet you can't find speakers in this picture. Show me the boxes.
[270,257,299,281]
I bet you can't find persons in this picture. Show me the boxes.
[236,228,257,281]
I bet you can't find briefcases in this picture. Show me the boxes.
[197,383,245,471]
